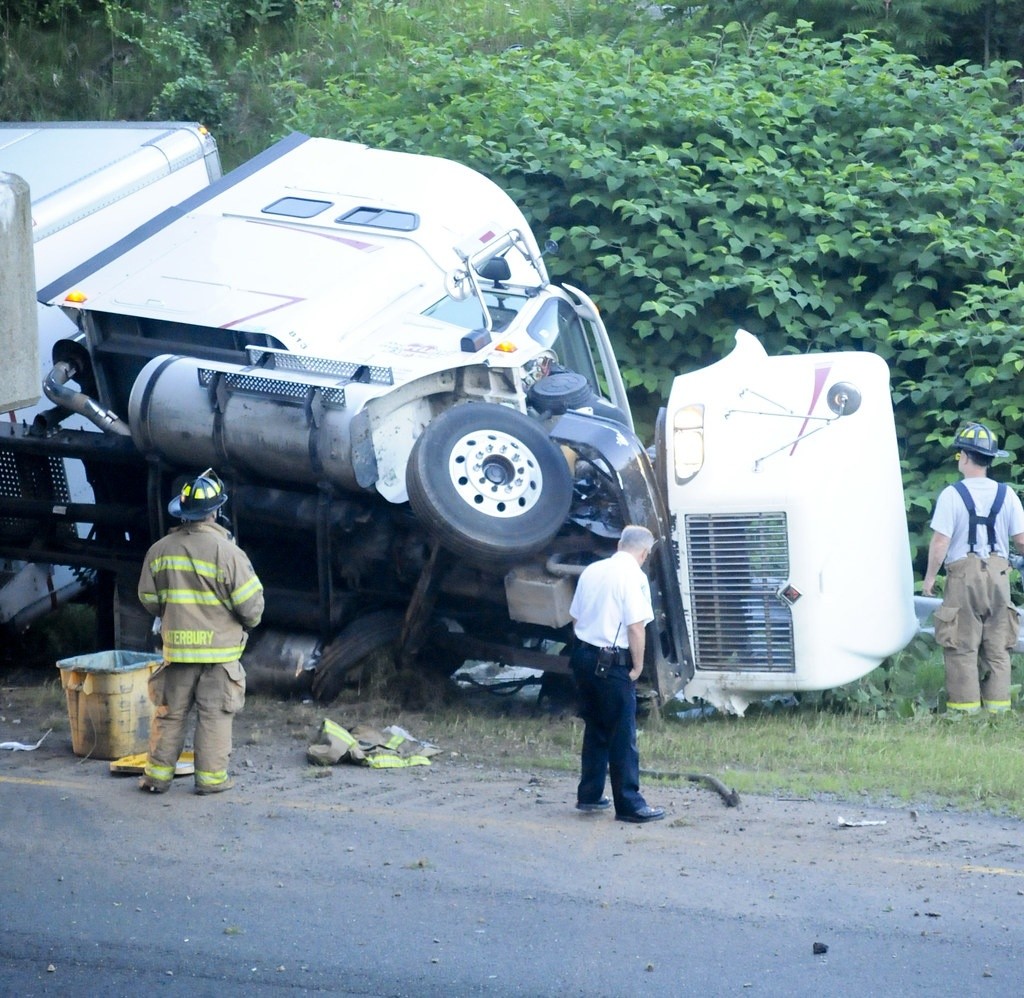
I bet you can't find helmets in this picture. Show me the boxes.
[168,475,228,520]
[950,423,1010,459]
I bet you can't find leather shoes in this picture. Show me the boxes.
[575,796,612,811]
[615,806,665,823]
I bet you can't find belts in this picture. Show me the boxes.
[575,640,601,652]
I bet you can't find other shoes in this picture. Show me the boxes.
[138,776,161,793]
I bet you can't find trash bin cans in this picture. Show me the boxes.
[54,648,167,761]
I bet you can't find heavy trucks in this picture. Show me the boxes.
[0,121,919,717]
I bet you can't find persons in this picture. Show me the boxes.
[139,468,266,794]
[568,525,665,823]
[922,423,1024,720]
